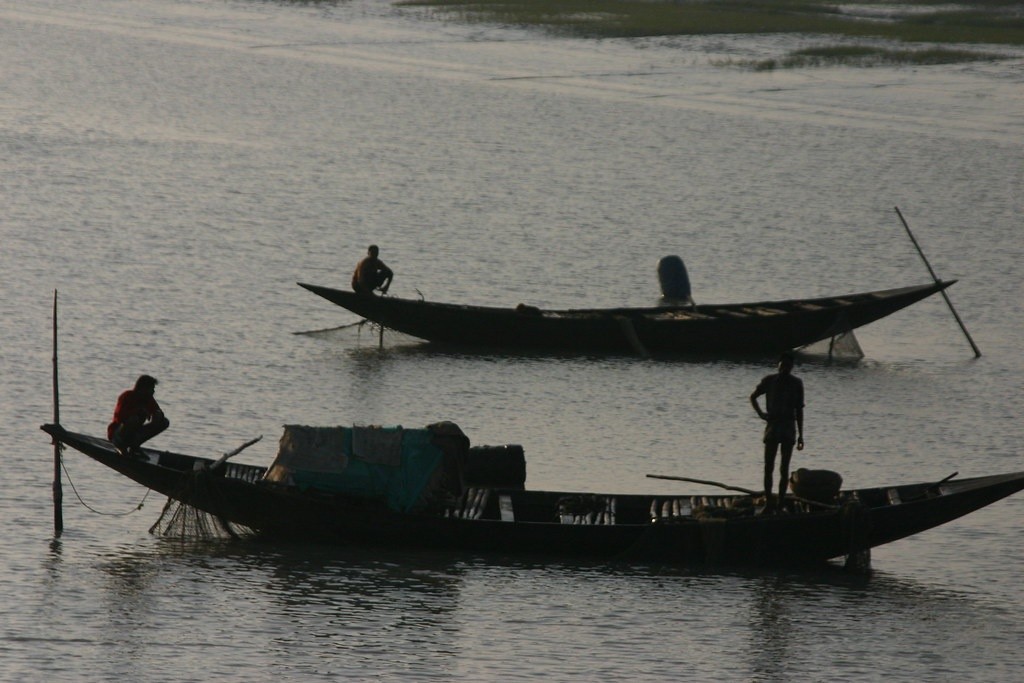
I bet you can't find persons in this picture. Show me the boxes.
[107,375,169,460]
[749,354,805,509]
[351,245,393,296]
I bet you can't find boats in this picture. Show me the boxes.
[293,277,963,353]
[41,416,1024,568]
